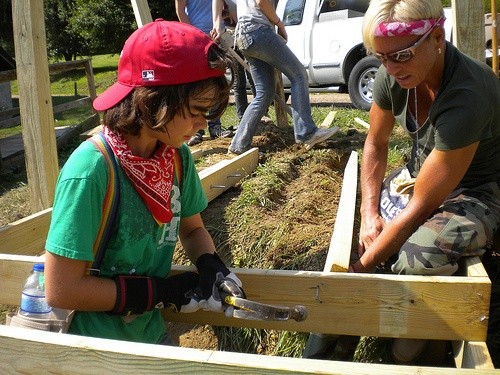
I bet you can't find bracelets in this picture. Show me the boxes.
[274,19,280,25]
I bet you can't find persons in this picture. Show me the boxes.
[351,0,500,363]
[44,18,246,342]
[175,0,339,157]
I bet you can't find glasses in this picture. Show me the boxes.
[371,15,443,63]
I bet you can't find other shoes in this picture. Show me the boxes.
[391,338,429,365]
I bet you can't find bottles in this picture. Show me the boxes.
[20,263,52,319]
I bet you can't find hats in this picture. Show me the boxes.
[92,18,227,111]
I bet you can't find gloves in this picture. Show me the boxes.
[196,253,246,312]
[114,268,198,316]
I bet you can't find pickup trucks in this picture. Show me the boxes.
[222,0,500,114]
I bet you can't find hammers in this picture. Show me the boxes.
[215,278,308,322]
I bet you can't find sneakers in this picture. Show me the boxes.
[228,150,263,160]
[188,132,202,147]
[208,120,233,141]
[304,126,340,150]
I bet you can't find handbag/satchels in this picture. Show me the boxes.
[4,300,75,334]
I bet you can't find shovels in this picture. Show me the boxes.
[213,30,344,150]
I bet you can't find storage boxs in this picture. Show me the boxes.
[318,9,364,22]
